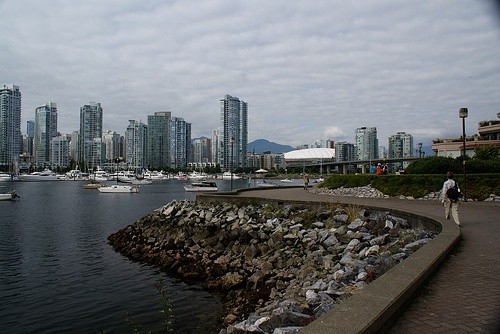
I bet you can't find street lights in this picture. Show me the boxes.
[418,142,425,158]
[459,108,468,202]
[230,135,236,191]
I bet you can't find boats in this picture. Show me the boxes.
[257,178,280,187]
[81,183,105,189]
[0,174,19,181]
[91,176,153,185]
[0,190,20,200]
[98,184,140,193]
[184,181,217,192]
[280,178,292,183]
[178,172,247,181]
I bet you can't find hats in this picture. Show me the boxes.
[378,163,381,165]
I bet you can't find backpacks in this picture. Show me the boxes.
[447,181,459,203]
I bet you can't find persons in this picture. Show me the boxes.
[303,171,310,191]
[398,165,404,175]
[371,161,388,175]
[442,172,462,228]
